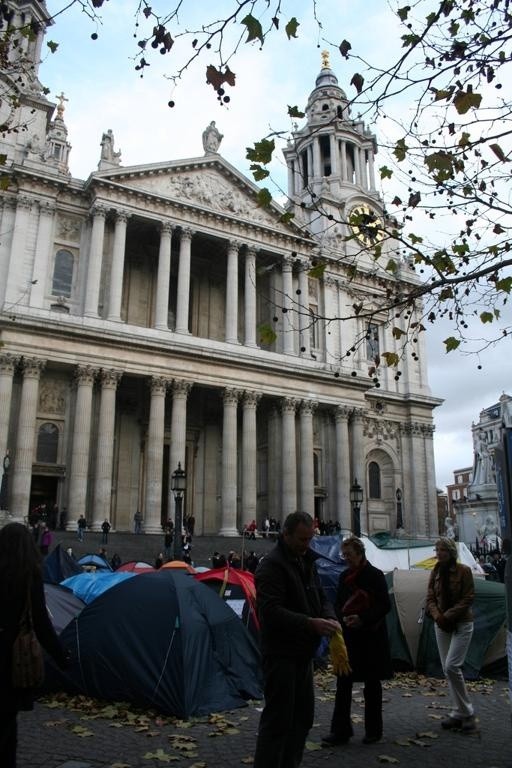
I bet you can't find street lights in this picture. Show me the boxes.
[349,476,365,539]
[170,461,188,561]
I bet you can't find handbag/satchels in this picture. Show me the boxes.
[8,628,47,698]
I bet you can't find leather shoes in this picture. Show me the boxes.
[321,730,351,743]
[361,732,383,745]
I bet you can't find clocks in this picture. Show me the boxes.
[347,205,386,248]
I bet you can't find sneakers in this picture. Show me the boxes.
[461,714,477,732]
[440,716,461,729]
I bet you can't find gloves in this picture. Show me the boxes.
[328,627,353,677]
